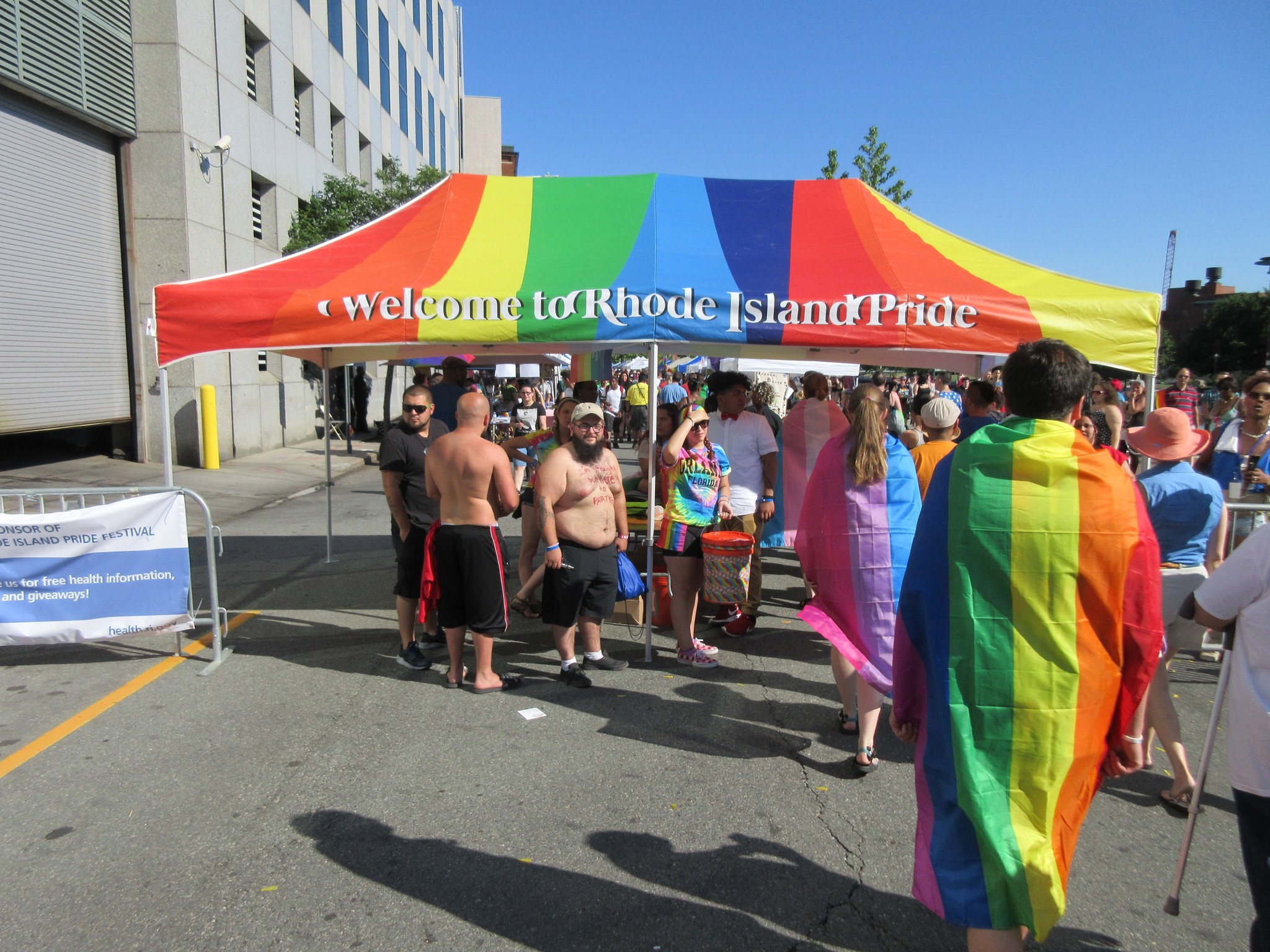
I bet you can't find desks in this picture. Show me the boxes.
[614,516,664,553]
[489,416,511,444]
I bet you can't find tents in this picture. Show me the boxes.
[155,171,1163,678]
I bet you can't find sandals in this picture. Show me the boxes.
[836,707,880,772]
[510,594,545,618]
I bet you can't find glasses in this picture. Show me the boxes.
[1177,374,1191,378]
[1090,390,1107,395]
[521,392,533,394]
[402,402,431,412]
[691,420,709,431]
[1218,386,1233,390]
[572,422,605,432]
[1248,391,1270,401]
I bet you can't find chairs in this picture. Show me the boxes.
[319,405,346,440]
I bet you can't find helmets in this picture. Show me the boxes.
[1215,372,1234,384]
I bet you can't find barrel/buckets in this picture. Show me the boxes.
[648,566,676,626]
[701,511,755,605]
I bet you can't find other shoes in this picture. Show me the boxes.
[1160,779,1205,815]
[612,434,638,448]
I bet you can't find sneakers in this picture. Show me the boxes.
[397,624,448,671]
[558,655,628,688]
[708,603,756,637]
[673,639,718,667]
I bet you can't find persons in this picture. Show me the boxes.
[793,381,920,776]
[888,336,1165,952]
[1121,397,1223,817]
[1193,510,1270,952]
[373,349,1268,699]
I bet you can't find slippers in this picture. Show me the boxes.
[445,665,524,695]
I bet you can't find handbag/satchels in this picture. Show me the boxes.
[617,551,649,600]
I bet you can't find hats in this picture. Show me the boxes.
[921,397,960,428]
[609,376,618,381]
[1133,380,1145,388]
[571,403,604,421]
[1126,407,1211,461]
[442,356,471,368]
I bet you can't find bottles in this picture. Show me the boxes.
[493,412,497,421]
[1240,454,1256,490]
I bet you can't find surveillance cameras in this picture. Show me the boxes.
[214,135,232,151]
